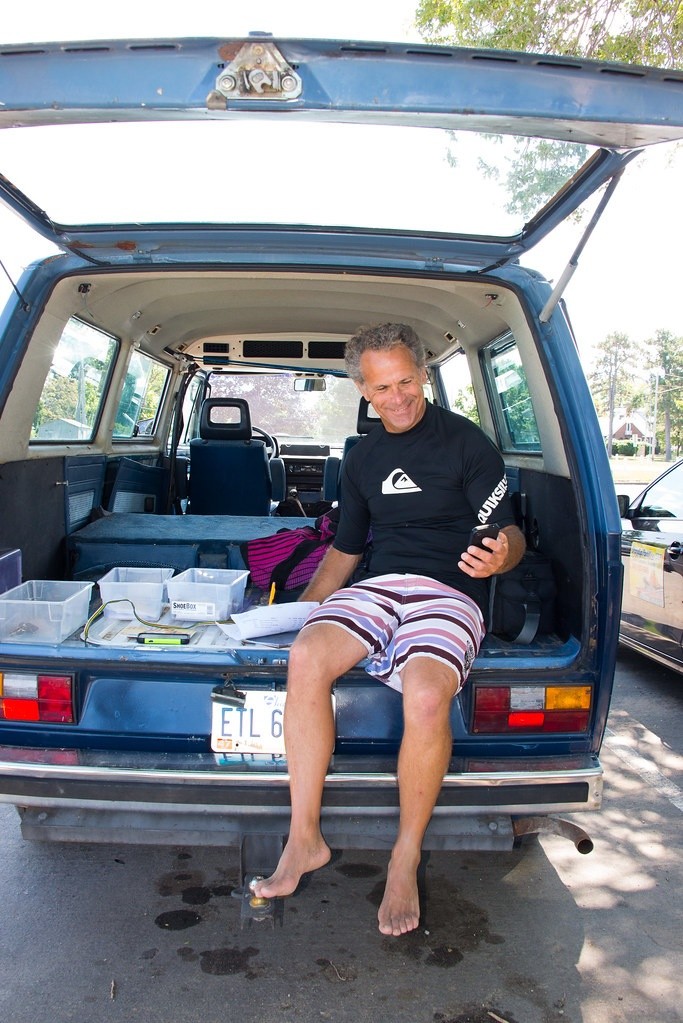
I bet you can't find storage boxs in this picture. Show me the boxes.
[1,581,96,648]
[163,568,250,621]
[97,567,175,622]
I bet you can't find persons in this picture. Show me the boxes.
[255,321,528,936]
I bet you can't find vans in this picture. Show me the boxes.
[0,30,682,859]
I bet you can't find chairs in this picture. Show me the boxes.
[175,397,288,515]
[322,397,381,507]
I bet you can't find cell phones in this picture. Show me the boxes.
[466,522,500,569]
[136,633,190,644]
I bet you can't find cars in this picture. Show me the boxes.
[614,459,683,684]
